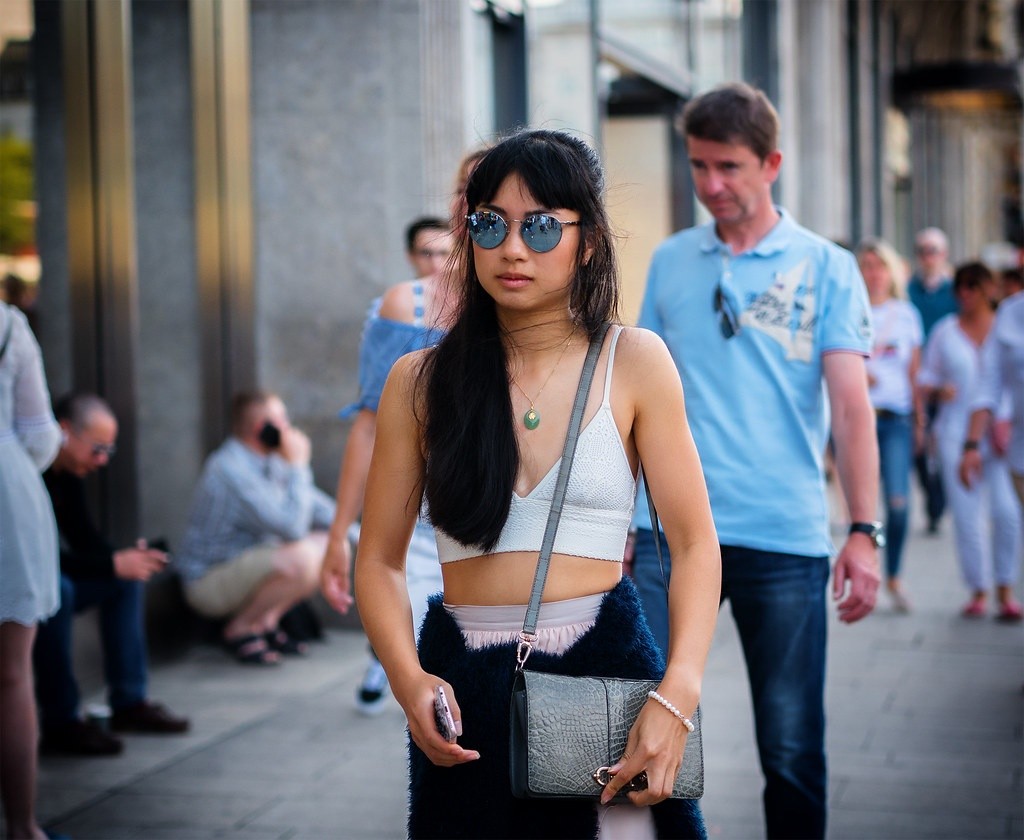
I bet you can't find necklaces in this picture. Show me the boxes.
[505,318,575,430]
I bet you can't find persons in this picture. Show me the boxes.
[0,291,63,840]
[33,390,194,758]
[354,213,458,718]
[628,88,889,840]
[353,129,723,838]
[175,390,362,667]
[318,145,497,642]
[821,224,1024,622]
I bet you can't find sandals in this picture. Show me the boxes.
[223,626,281,668]
[264,629,310,654]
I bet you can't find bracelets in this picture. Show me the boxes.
[964,440,979,450]
[642,689,697,734]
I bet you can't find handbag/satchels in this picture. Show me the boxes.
[510,669,703,801]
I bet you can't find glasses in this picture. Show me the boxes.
[462,213,586,254]
[65,432,116,458]
[711,283,737,340]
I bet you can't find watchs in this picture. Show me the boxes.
[845,522,886,549]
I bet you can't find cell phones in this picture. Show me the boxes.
[259,422,279,449]
[434,686,456,744]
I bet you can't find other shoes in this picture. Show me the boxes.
[884,578,912,615]
[39,720,121,759]
[357,660,393,715]
[965,594,986,620]
[999,597,1024,619]
[104,696,190,731]
